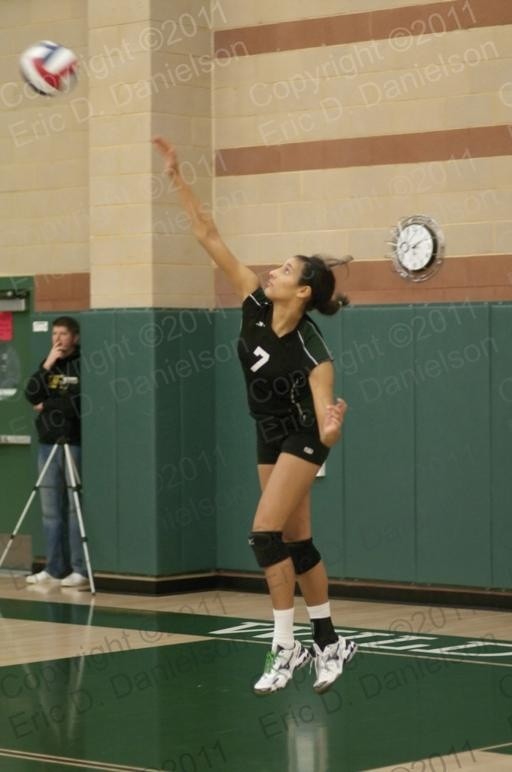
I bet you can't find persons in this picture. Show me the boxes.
[22,315,89,587]
[150,133,360,694]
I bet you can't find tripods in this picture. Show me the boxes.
[0,437,96,596]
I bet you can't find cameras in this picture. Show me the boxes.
[58,376,79,390]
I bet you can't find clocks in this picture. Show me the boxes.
[385,214,446,284]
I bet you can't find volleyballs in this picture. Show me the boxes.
[19,39,80,98]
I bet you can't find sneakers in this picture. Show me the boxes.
[309,635,357,694]
[60,573,91,586]
[26,571,60,583]
[254,640,313,694]
